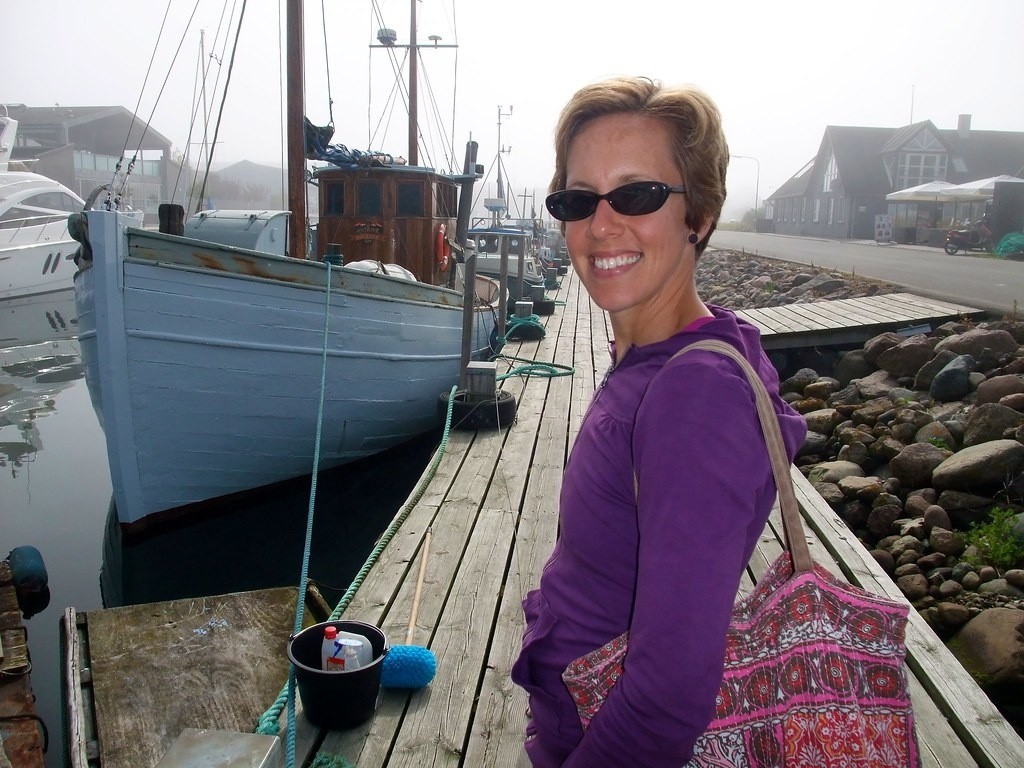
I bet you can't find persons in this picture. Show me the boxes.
[510,76,808,767]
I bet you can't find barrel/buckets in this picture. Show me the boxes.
[286,620,391,732]
[321,626,373,672]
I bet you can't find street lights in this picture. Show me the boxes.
[733,155,760,231]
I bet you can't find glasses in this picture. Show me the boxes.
[544,181,687,221]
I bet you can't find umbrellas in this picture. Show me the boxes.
[941,174,1024,195]
[886,180,975,201]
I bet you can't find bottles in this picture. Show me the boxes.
[344,649,361,673]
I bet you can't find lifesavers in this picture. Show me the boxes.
[435,223,449,271]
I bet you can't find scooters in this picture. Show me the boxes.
[945,211,996,257]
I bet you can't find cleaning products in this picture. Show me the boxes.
[320,626,375,672]
[334,637,364,673]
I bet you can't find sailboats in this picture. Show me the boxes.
[0,0,568,530]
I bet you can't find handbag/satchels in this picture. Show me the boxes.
[564,340,919,768]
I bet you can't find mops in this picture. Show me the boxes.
[379,522,436,693]
